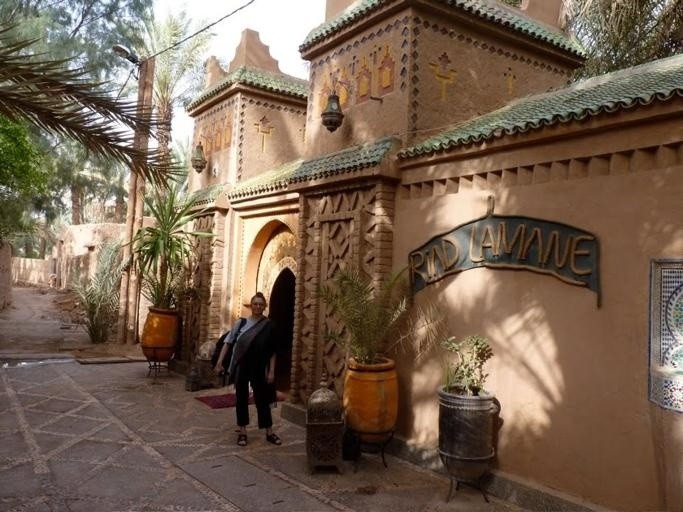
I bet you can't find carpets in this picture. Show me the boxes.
[194,392,284,409]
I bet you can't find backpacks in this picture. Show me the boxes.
[210,317,246,378]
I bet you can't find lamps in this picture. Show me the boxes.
[321,76,353,134]
[189,139,206,174]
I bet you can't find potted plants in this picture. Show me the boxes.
[129,261,208,361]
[434,332,495,479]
[60,236,130,343]
[303,258,450,441]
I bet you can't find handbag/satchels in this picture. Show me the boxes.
[237,434,247,446]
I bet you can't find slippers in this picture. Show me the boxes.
[266,434,282,445]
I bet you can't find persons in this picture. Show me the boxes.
[212,291,284,446]
